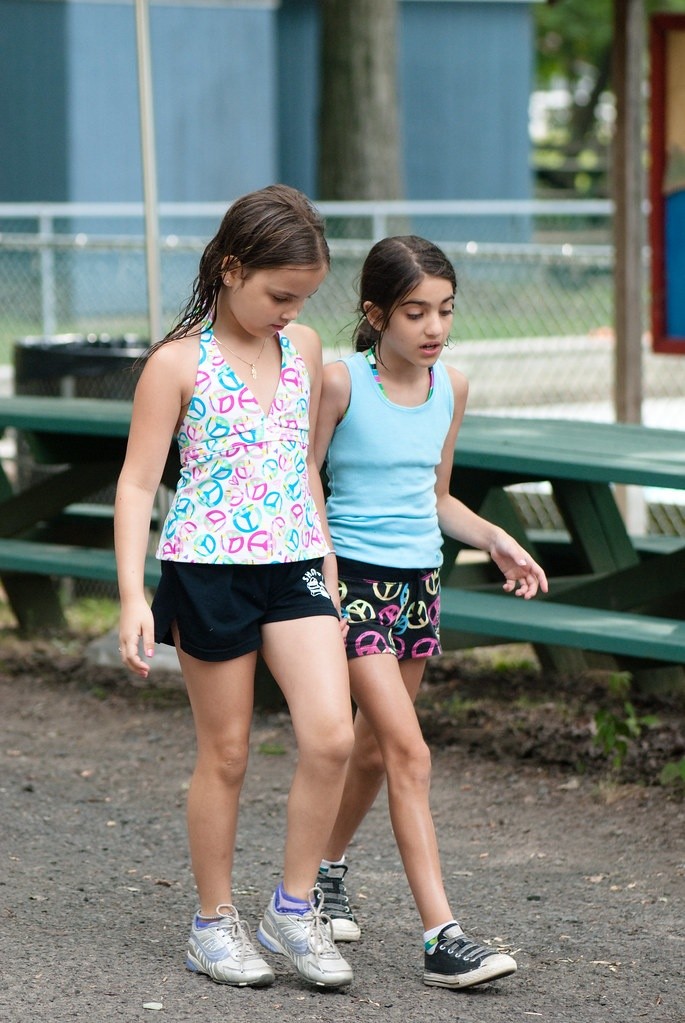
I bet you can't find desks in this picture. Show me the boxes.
[0,397,685,702]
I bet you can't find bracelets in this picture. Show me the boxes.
[328,550,336,554]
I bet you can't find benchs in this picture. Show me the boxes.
[63,502,684,564]
[0,540,684,661]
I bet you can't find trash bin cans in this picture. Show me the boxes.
[14,335,150,599]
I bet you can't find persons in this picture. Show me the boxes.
[314,236,549,989]
[115,181,358,988]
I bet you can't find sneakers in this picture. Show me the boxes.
[256,887,354,987]
[186,904,275,986]
[313,866,362,941]
[421,923,517,989]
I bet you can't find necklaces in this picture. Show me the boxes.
[213,336,268,379]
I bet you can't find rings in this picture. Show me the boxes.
[118,647,123,653]
